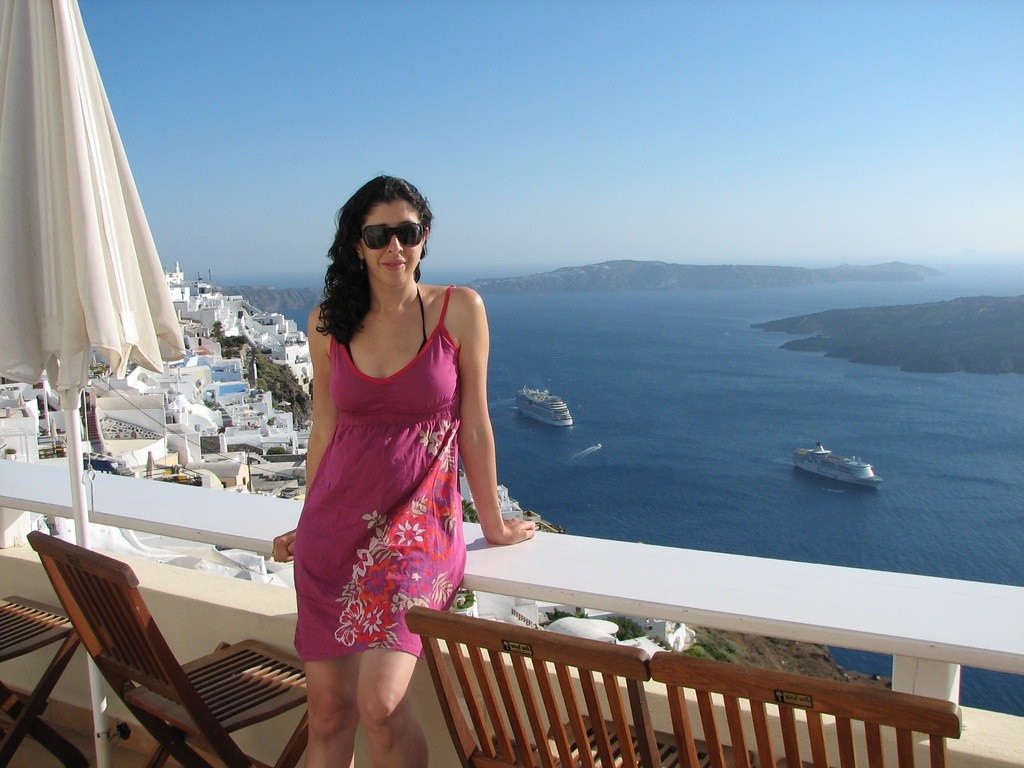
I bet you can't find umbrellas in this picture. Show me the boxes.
[0,0,187,768]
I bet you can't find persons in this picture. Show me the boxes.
[273,176,535,768]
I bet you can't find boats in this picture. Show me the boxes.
[793,441,883,487]
[516,385,573,426]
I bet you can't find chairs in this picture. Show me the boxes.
[402,604,963,768]
[27,531,311,768]
[0,595,93,768]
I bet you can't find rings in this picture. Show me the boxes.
[272,551,276,555]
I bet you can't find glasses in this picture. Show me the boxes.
[360,223,426,249]
